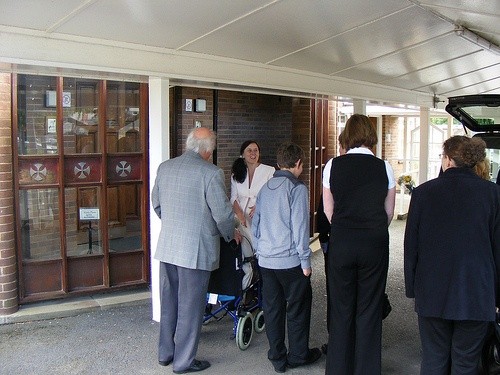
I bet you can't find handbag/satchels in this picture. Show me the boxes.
[480,317,500,375]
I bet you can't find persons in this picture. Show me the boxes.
[229,140,276,235]
[151,128,243,372]
[316,131,347,356]
[404,135,500,374]
[323,113,395,375]
[252,142,321,373]
[224,211,262,295]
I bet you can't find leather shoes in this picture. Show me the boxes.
[173,359,210,374]
[286,348,322,369]
[274,356,287,373]
[159,359,173,366]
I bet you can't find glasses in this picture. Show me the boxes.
[439,154,452,161]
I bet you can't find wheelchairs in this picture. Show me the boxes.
[202,237,266,350]
